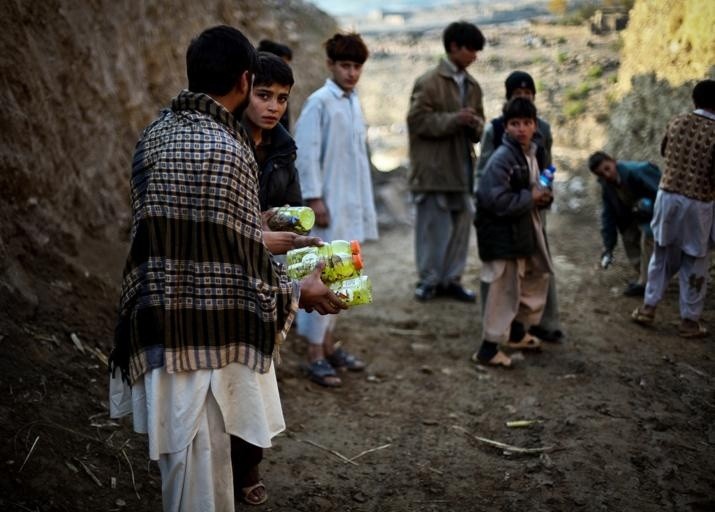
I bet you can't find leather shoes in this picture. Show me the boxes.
[414,281,476,303]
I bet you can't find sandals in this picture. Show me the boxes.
[301,348,367,389]
[235,480,268,506]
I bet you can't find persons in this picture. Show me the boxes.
[252,40,293,129]
[632,79,715,337]
[294,33,380,387]
[479,71,564,343]
[406,19,486,307]
[230,52,303,504]
[472,98,556,369]
[589,151,663,295]
[108,24,349,511]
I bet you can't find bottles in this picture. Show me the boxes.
[601,253,611,269]
[268,207,315,232]
[636,199,651,210]
[540,164,558,187]
[288,240,374,306]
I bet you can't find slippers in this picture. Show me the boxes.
[473,349,513,370]
[504,332,542,352]
[677,322,707,338]
[631,306,654,324]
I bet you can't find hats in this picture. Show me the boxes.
[506,72,534,98]
